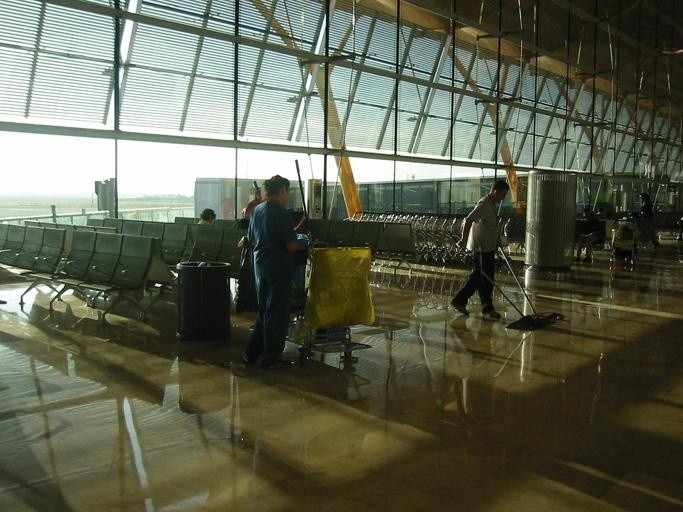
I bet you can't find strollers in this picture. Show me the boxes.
[608,212,640,265]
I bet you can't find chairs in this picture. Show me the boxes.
[1,217,250,323]
[574,218,606,259]
[305,211,525,277]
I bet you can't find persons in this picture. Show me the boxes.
[246,174,305,369]
[195,208,217,226]
[574,207,603,265]
[451,180,510,321]
[635,192,662,251]
[234,200,314,234]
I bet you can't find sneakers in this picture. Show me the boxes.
[451,299,470,316]
[262,358,296,369]
[480,310,500,320]
[242,350,253,363]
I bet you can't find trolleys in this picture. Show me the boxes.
[249,228,374,364]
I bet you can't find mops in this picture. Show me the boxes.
[455,242,564,331]
